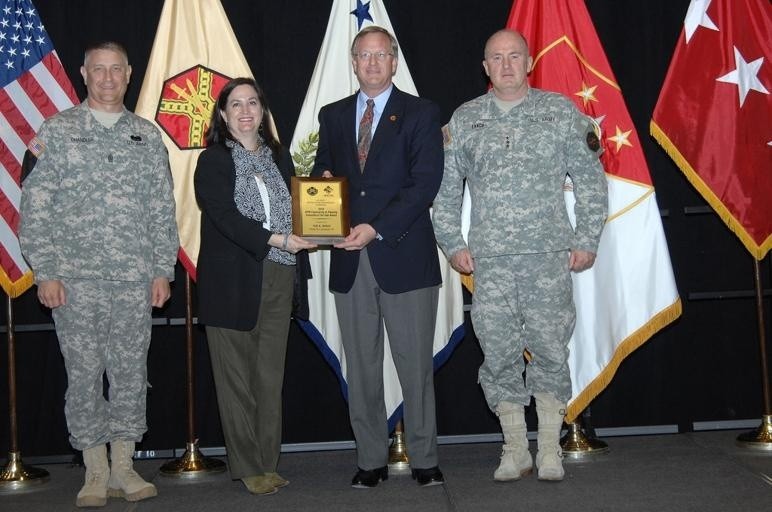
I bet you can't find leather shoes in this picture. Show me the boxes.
[413,467,444,487]
[351,465,388,487]
[240,475,278,497]
[265,472,290,487]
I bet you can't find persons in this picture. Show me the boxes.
[431,30,608,482]
[189,77,320,497]
[18,38,181,508]
[310,27,445,485]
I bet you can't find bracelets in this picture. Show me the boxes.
[281,233,289,251]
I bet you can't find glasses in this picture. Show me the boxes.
[352,50,393,59]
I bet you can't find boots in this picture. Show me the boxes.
[108,439,158,502]
[75,443,110,507]
[533,392,566,481]
[494,401,533,482]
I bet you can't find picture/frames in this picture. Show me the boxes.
[292,176,350,245]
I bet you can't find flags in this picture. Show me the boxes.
[288,2,469,437]
[0,0,84,301]
[459,2,682,425]
[132,2,281,284]
[651,1,772,262]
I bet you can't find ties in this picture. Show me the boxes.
[357,99,375,174]
